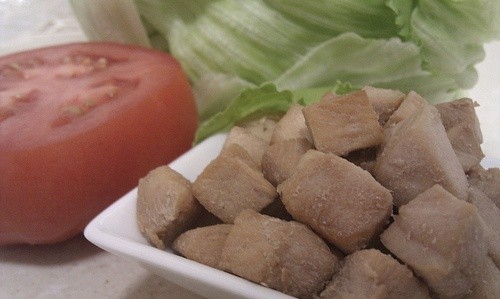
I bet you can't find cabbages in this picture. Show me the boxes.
[67,0,500,146]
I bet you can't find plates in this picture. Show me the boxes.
[84,38,498,299]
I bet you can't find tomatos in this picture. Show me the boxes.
[0,42,198,245]
